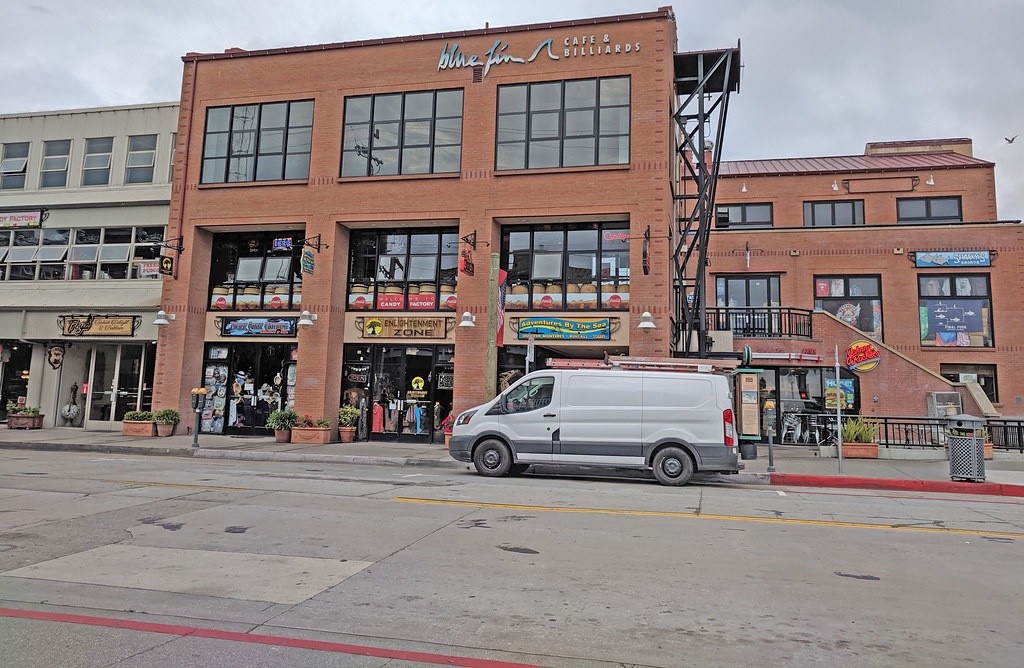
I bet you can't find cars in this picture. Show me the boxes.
[783,399,830,431]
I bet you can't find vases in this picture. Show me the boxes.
[443,432,453,450]
[291,427,331,445]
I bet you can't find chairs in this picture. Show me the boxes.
[781,412,819,445]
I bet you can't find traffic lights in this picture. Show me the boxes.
[800,391,808,399]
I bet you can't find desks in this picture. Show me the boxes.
[812,425,844,446]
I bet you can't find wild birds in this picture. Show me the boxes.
[1005,135,1018,144]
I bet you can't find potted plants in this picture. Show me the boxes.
[940,428,994,462]
[336,403,361,443]
[155,409,182,438]
[122,411,158,438]
[841,407,882,459]
[6,399,45,430]
[265,409,299,443]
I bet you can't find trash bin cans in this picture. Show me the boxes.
[944,414,988,482]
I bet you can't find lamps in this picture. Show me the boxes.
[831,180,838,191]
[926,175,935,185]
[741,183,747,193]
[297,310,318,330]
[152,310,175,329]
[458,312,476,331]
[637,311,657,333]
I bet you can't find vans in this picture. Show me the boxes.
[447,367,746,486]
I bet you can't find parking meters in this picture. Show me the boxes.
[190,386,207,446]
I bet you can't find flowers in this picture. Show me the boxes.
[292,414,331,428]
[442,414,456,432]
